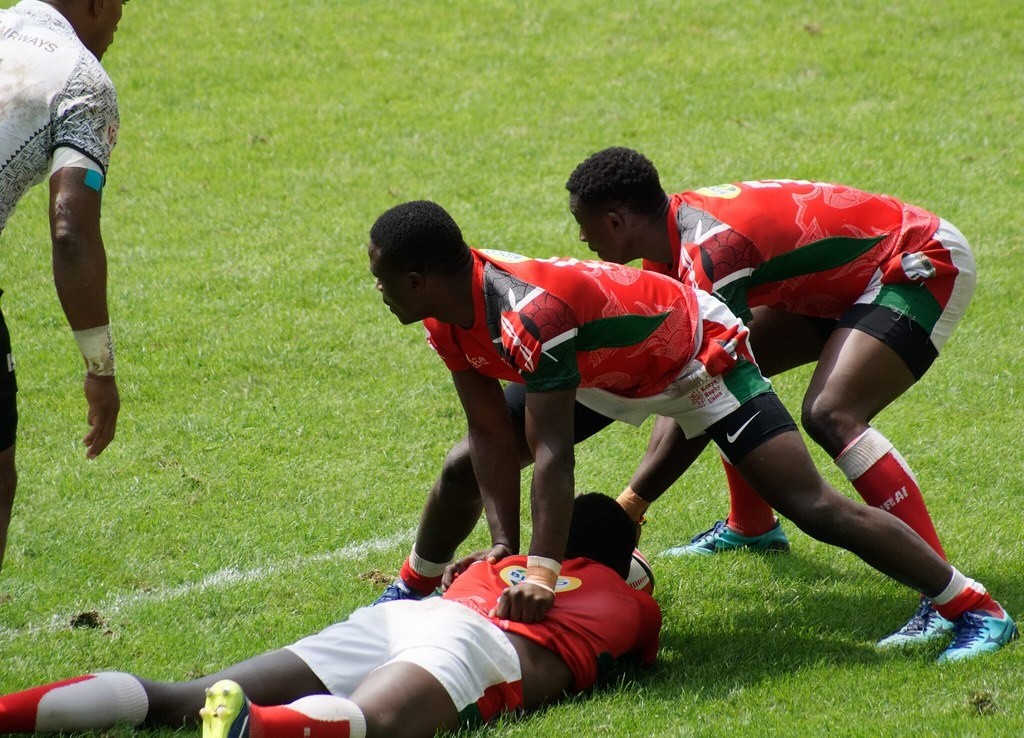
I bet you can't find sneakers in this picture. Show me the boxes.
[370,579,443,604]
[878,598,954,648]
[655,515,789,557]
[200,679,251,738]
[938,601,1019,662]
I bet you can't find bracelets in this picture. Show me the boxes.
[616,486,650,524]
[74,322,115,380]
[525,556,563,596]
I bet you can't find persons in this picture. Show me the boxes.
[0,493,661,738]
[565,147,976,651]
[368,201,1018,664]
[0,0,134,553]
